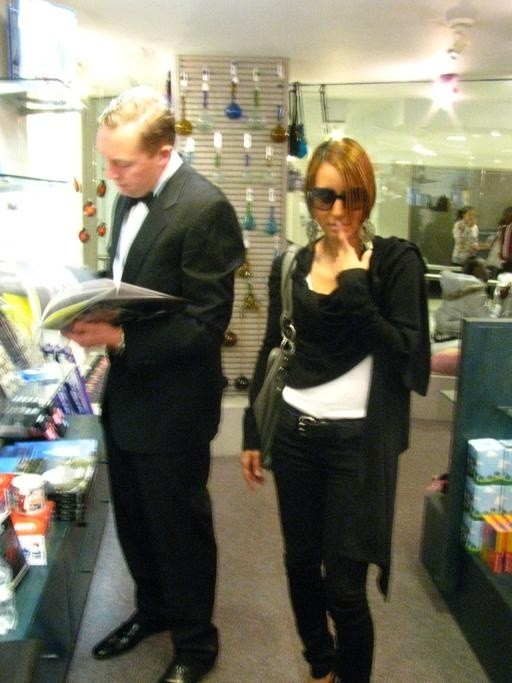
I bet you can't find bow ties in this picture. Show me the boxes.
[126,192,153,210]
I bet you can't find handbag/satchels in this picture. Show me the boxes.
[253,241,307,470]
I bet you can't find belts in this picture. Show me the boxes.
[277,407,368,439]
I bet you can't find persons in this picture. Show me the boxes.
[487,207,512,270]
[452,206,480,266]
[433,255,510,342]
[60,86,244,683]
[242,137,431,683]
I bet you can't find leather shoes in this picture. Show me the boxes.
[158,661,195,683]
[93,617,154,659]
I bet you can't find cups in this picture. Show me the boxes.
[10,473,47,516]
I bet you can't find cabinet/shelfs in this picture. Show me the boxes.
[0,412,110,683]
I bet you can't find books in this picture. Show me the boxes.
[0,292,99,528]
[34,270,191,329]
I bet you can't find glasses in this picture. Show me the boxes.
[307,187,368,211]
